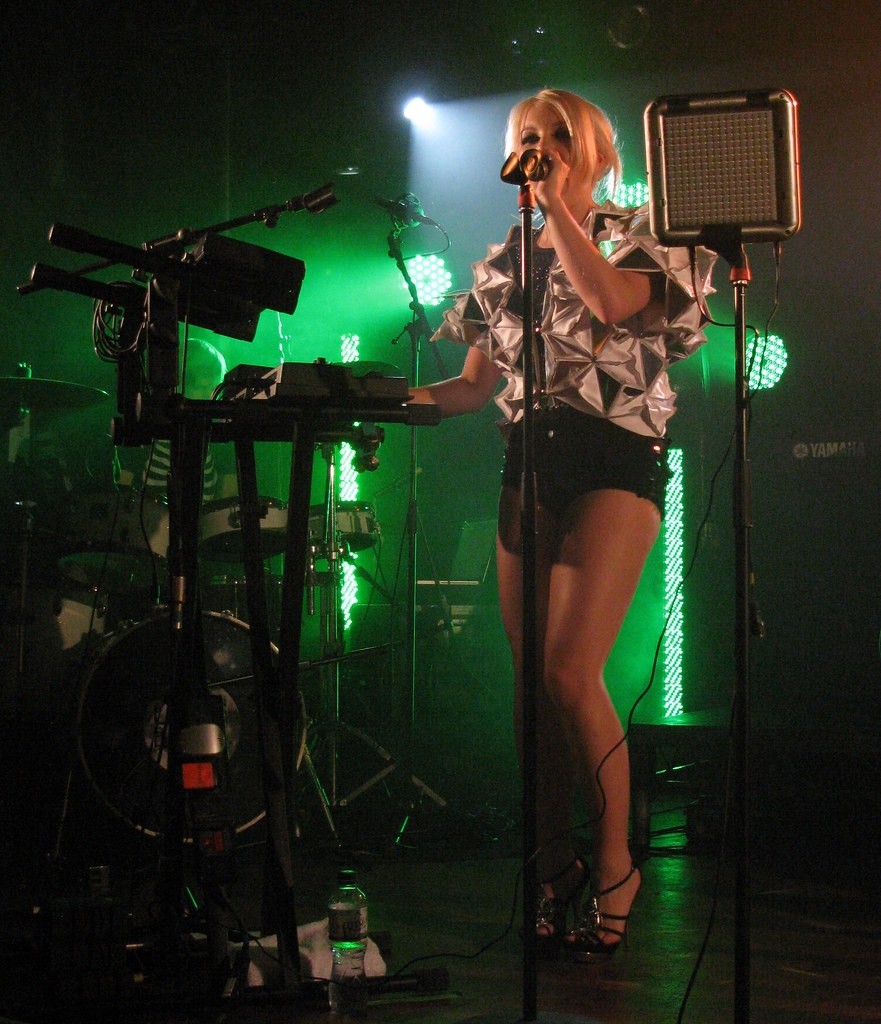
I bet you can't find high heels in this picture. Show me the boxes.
[564,865,642,963]
[532,851,591,939]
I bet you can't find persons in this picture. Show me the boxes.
[406,90,718,963]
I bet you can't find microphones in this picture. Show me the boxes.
[529,159,549,181]
[378,193,435,230]
[110,439,122,482]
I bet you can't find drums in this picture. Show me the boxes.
[308,500,379,560]
[24,602,311,844]
[1,580,110,723]
[200,493,289,557]
[57,482,177,600]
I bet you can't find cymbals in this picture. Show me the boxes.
[0,358,110,414]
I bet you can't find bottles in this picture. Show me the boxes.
[327,870,369,1012]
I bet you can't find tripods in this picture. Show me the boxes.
[305,443,448,816]
